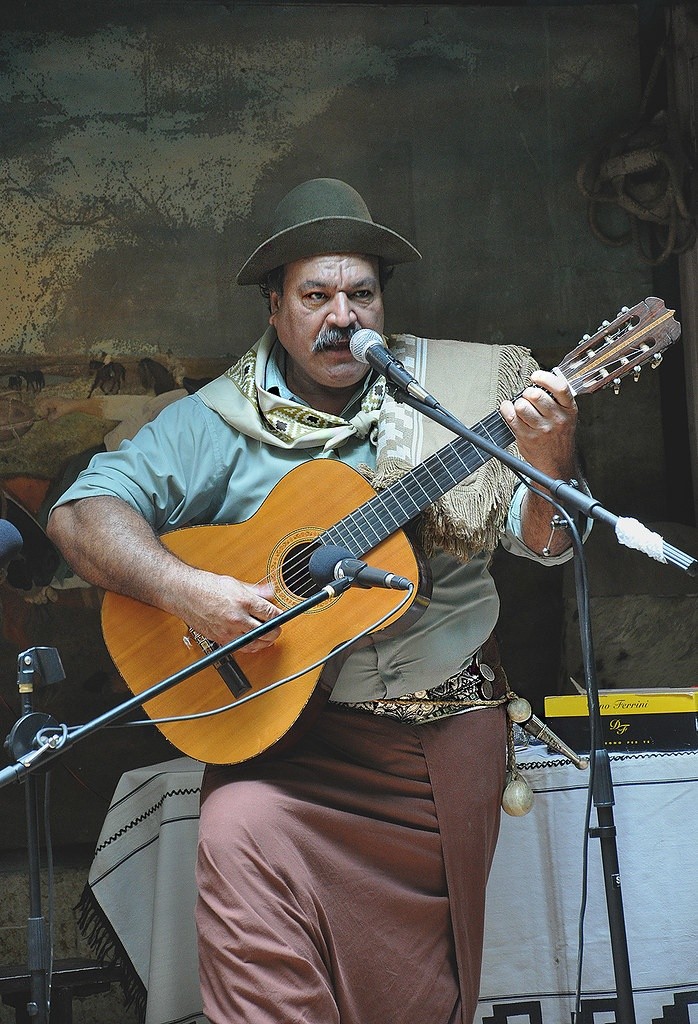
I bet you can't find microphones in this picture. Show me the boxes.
[349,328,440,409]
[308,544,415,591]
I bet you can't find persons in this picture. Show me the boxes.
[47,179,594,1024]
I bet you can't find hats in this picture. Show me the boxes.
[236,178,422,286]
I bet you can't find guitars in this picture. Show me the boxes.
[97,294,683,770]
